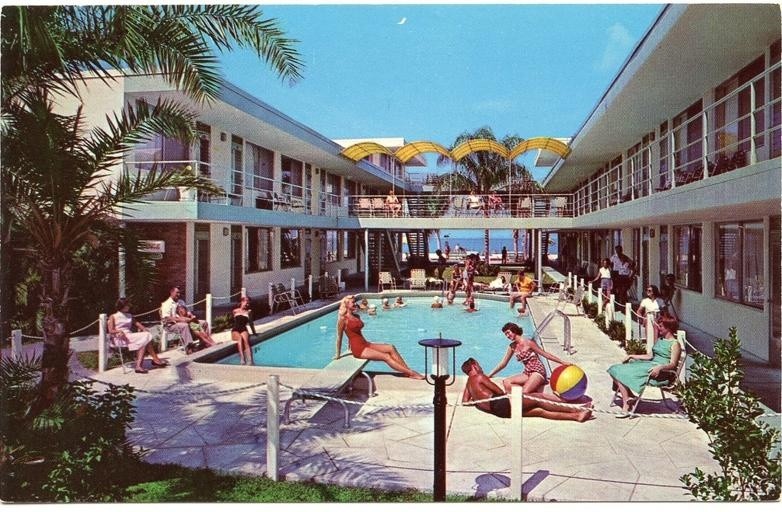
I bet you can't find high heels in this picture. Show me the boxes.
[626,395,639,407]
[615,408,635,420]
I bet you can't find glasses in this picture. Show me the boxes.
[645,288,653,293]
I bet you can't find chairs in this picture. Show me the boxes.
[555,288,586,314]
[449,151,747,218]
[282,350,376,429]
[164,174,398,217]
[157,306,188,351]
[610,334,687,418]
[271,265,513,316]
[109,332,142,374]
[649,288,679,320]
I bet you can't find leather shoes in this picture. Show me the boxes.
[151,359,167,367]
[135,367,150,374]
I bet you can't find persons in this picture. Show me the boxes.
[106,297,165,374]
[406,241,481,313]
[606,315,682,419]
[461,358,594,423]
[160,285,208,355]
[174,305,222,347]
[488,189,503,214]
[553,244,636,314]
[385,191,402,218]
[636,273,675,339]
[500,246,549,313]
[231,296,258,366]
[333,296,426,380]
[359,296,406,315]
[466,190,486,215]
[485,322,576,403]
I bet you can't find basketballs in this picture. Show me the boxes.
[550,365,587,400]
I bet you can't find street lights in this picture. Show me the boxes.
[443,234,450,256]
